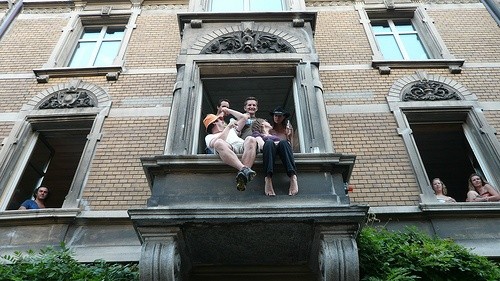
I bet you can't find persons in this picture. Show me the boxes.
[18,186,50,211]
[236,97,262,140]
[466,190,484,201]
[251,118,282,151]
[469,173,500,202]
[432,178,457,203]
[203,107,258,191]
[207,99,231,154]
[262,106,299,196]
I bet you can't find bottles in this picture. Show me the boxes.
[286,119,291,135]
[310,139,319,153]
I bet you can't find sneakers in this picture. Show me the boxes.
[241,166,255,181]
[235,171,246,192]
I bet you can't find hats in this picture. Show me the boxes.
[270,106,290,119]
[203,112,224,131]
